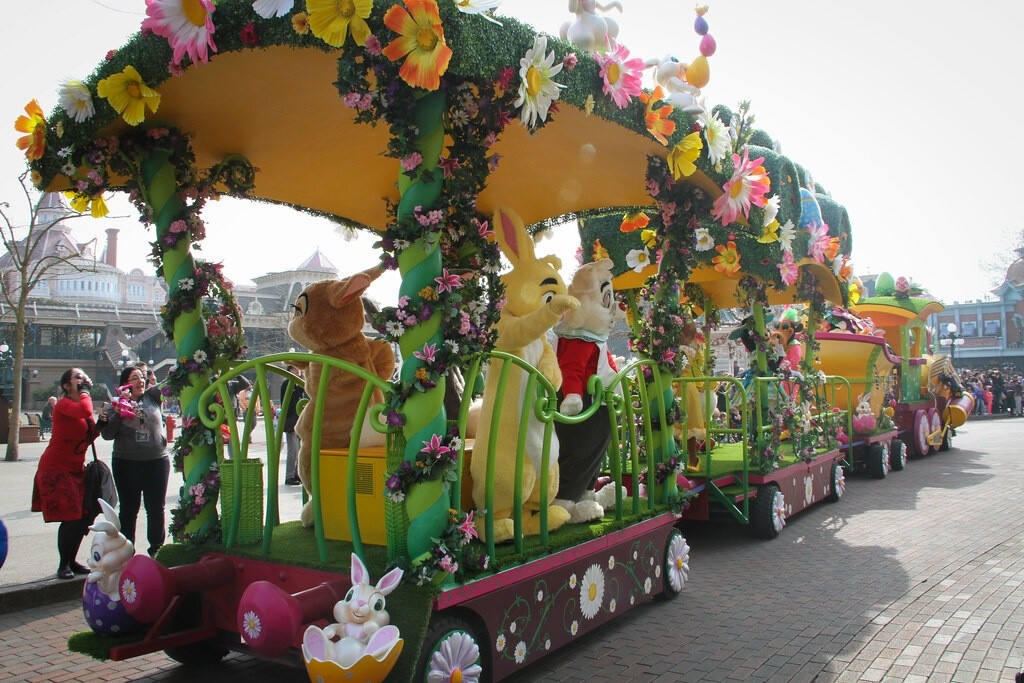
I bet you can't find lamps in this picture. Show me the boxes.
[33,370,38,378]
[148,359,153,367]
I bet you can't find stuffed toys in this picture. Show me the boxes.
[461,204,572,544]
[288,260,397,529]
[853,391,876,432]
[547,257,628,522]
[666,317,807,471]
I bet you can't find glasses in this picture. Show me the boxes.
[128,374,146,381]
[70,372,83,378]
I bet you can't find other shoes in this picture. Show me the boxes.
[57,566,74,578]
[72,562,90,575]
[285,478,300,485]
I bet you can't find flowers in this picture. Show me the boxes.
[16,0,906,591]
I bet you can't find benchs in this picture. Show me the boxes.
[25,413,52,439]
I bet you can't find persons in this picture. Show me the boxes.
[268,400,283,435]
[280,364,309,486]
[237,380,260,444]
[954,366,1024,418]
[42,395,58,422]
[101,366,180,557]
[212,362,251,459]
[134,361,167,446]
[31,368,109,579]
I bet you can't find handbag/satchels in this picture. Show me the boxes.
[85,460,118,515]
